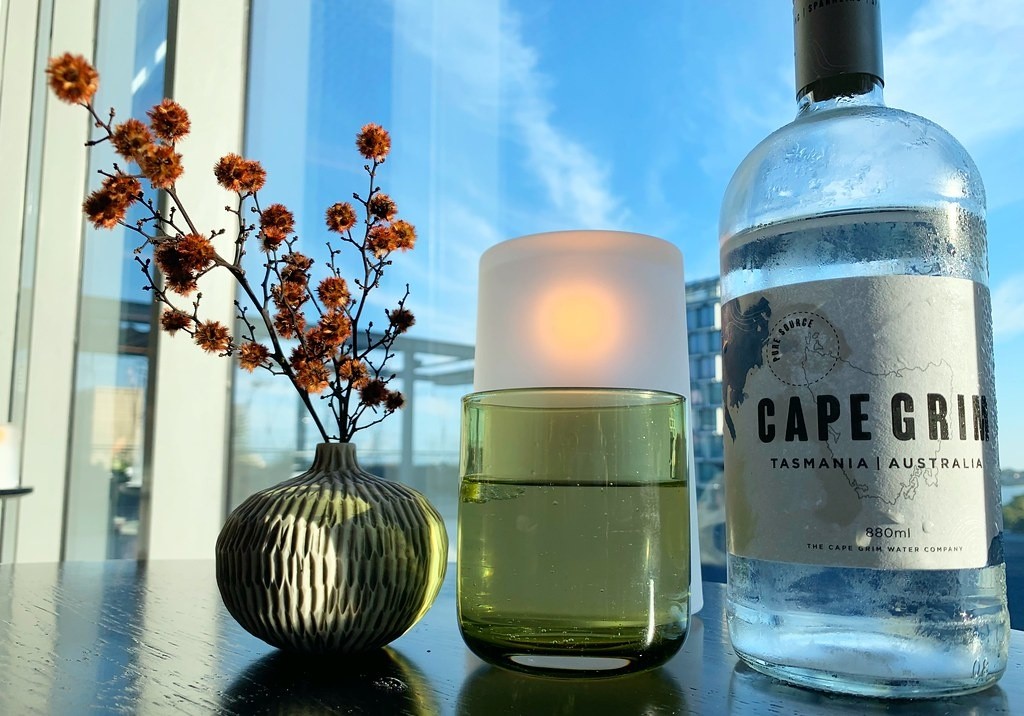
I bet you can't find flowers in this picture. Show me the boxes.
[46,49,419,442]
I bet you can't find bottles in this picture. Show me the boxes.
[717,0,1012,699]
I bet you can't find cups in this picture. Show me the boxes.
[454,386,695,684]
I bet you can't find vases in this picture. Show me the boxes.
[215,442,449,658]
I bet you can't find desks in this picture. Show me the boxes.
[0,559,1024,716]
